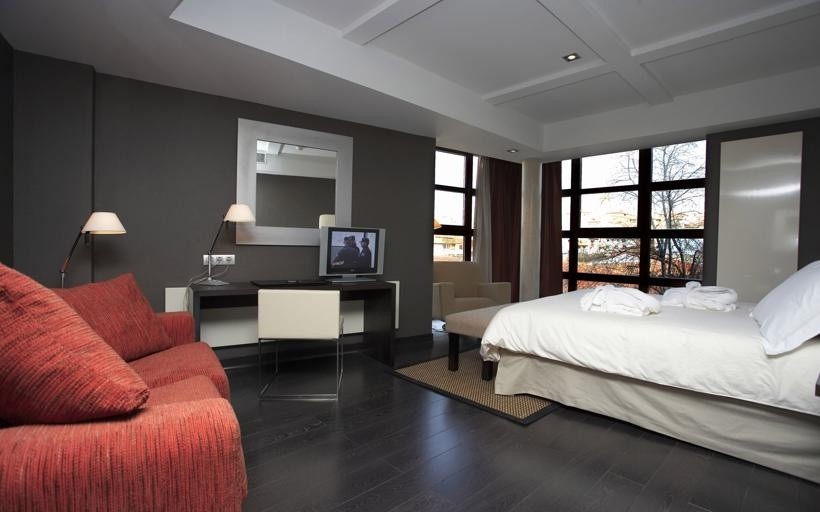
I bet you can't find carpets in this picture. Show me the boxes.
[385,347,562,426]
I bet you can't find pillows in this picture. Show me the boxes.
[0,263,151,423]
[49,272,175,362]
[750,261,820,356]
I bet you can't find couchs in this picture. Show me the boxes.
[432,261,511,322]
[1,312,247,512]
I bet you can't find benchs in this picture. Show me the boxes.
[445,300,521,381]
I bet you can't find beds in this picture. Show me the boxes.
[477,286,820,483]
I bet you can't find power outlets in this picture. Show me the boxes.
[203,254,236,265]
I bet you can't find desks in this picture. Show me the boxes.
[188,280,400,368]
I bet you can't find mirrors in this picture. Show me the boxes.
[236,118,354,247]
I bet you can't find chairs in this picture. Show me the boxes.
[257,289,345,403]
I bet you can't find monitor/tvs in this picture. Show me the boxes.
[319,225,386,284]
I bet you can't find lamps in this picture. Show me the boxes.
[60,211,126,289]
[192,204,255,286]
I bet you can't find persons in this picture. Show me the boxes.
[331,234,372,272]
